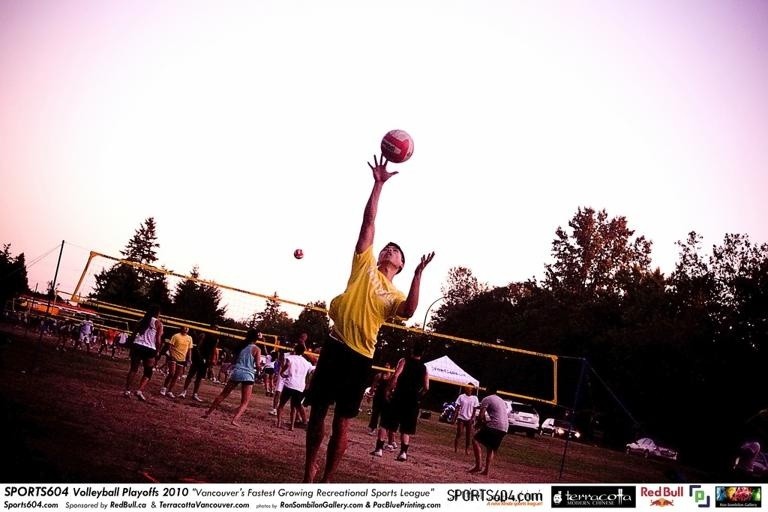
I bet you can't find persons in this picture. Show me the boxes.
[385,425,397,451]
[469,385,509,476]
[369,361,393,438]
[46,306,320,430]
[301,155,436,483]
[451,384,480,456]
[369,344,430,461]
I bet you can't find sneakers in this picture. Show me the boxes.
[150,365,229,386]
[383,441,397,452]
[125,389,146,401]
[370,449,383,456]
[269,410,278,416]
[168,391,176,398]
[160,387,167,396]
[192,393,203,402]
[178,390,187,398]
[397,453,407,460]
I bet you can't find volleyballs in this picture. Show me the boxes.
[294,249,304,259]
[381,131,414,162]
